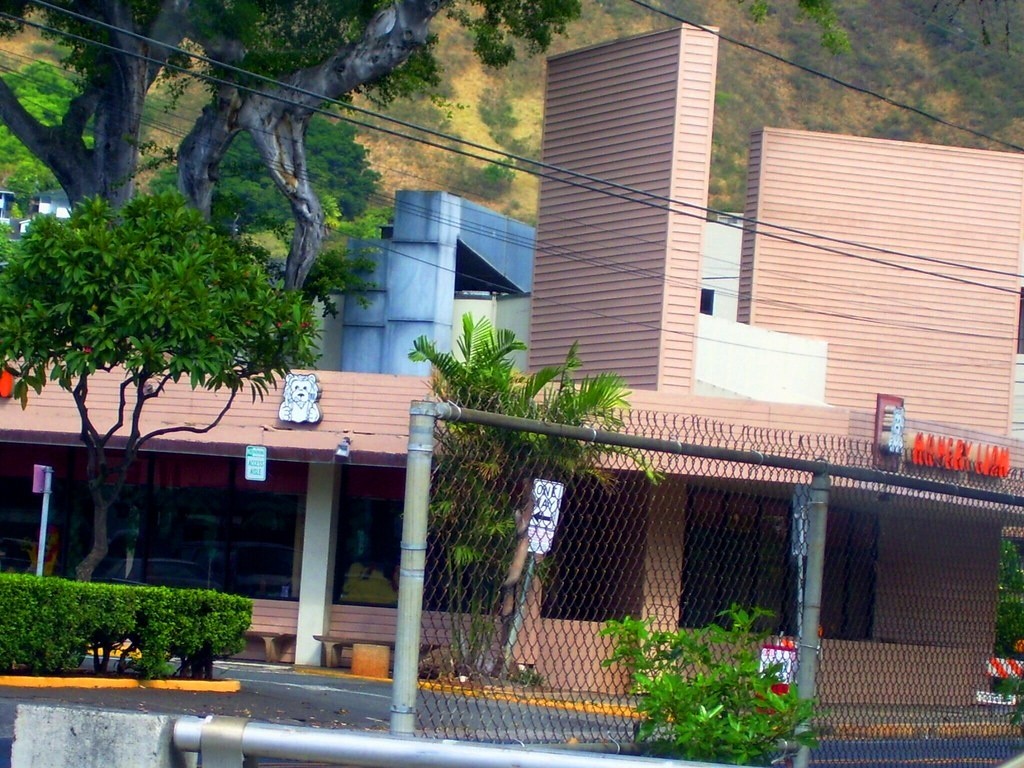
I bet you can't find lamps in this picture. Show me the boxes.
[334,437,351,457]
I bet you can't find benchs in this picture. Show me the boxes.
[244,630,296,663]
[313,635,440,677]
